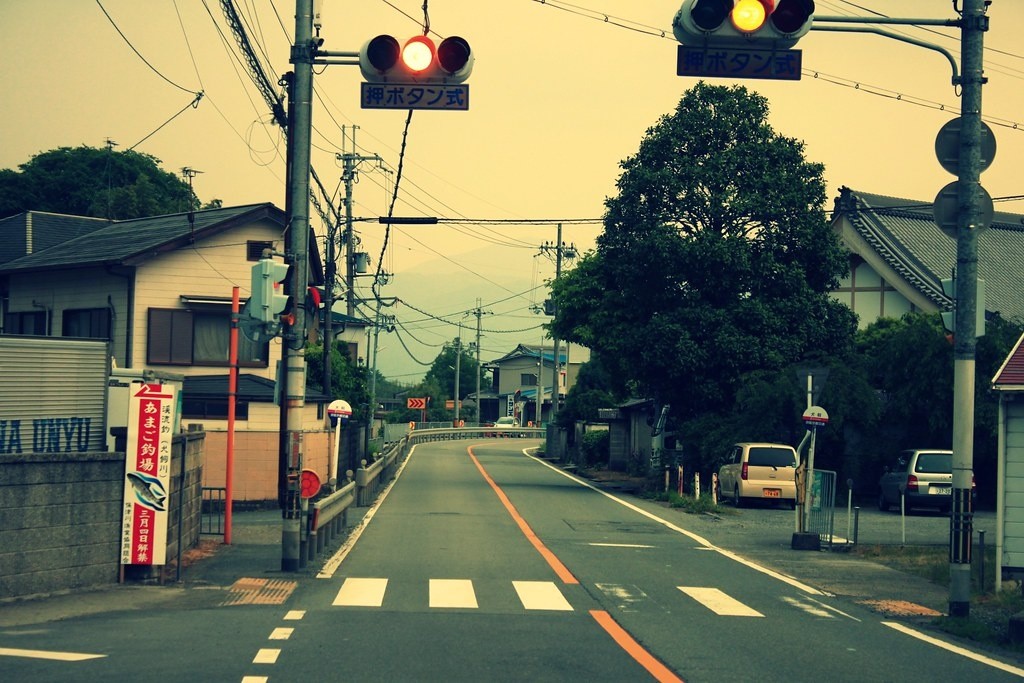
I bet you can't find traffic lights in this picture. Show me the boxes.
[250,260,294,325]
[359,36,472,76]
[678,0,813,41]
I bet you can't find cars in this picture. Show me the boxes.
[717,442,801,507]
[876,450,977,512]
[492,417,521,435]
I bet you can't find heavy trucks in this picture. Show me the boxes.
[649,405,688,473]
